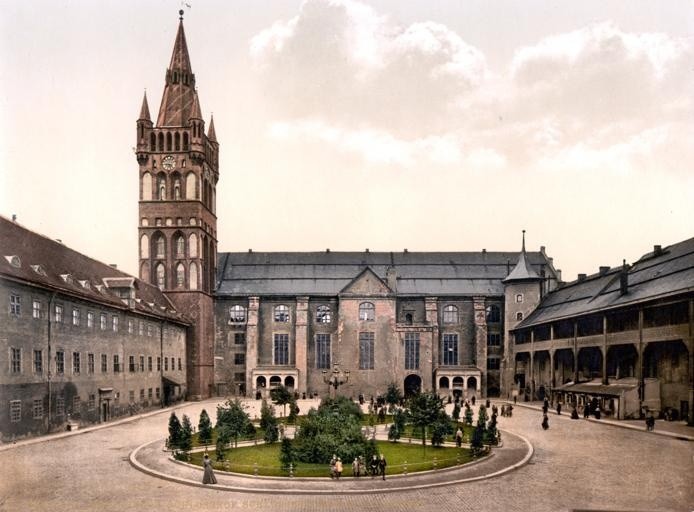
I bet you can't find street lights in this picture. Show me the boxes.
[322,363,350,399]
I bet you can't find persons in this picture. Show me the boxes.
[646,414,655,430]
[256,391,261,400]
[540,397,601,429]
[293,391,314,400]
[358,393,385,423]
[203,453,217,484]
[329,453,387,481]
[447,391,516,447]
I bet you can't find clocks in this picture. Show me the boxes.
[162,156,177,172]
